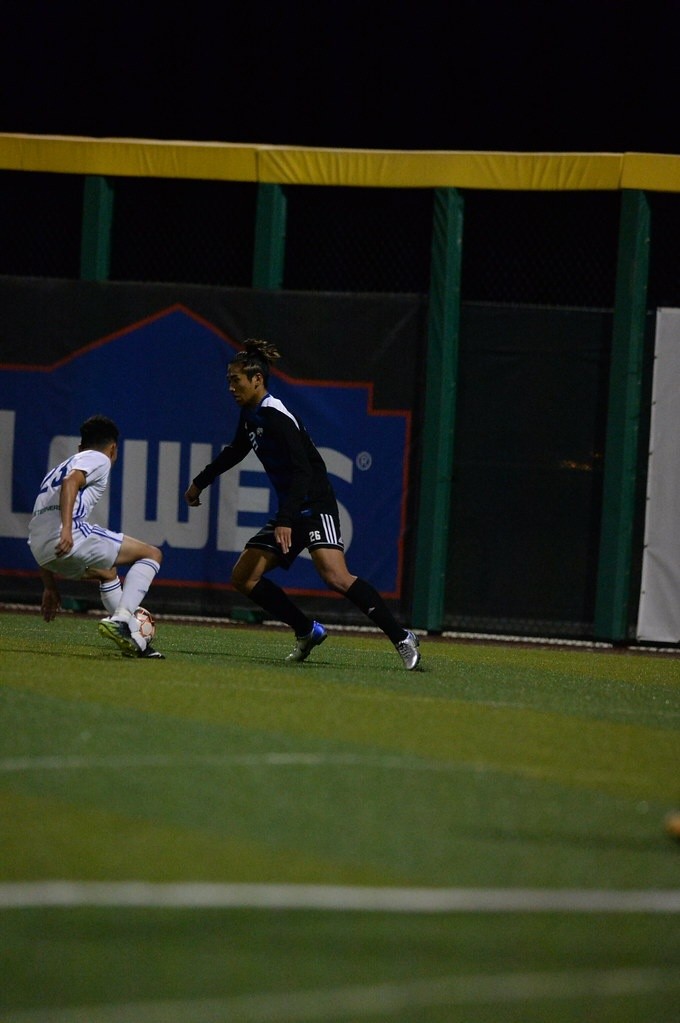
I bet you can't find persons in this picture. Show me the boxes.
[29,417,167,659]
[185,339,422,670]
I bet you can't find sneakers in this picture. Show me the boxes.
[99,616,146,657]
[121,642,165,658]
[285,621,327,661]
[394,628,421,671]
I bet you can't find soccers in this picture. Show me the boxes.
[133,606,156,646]
